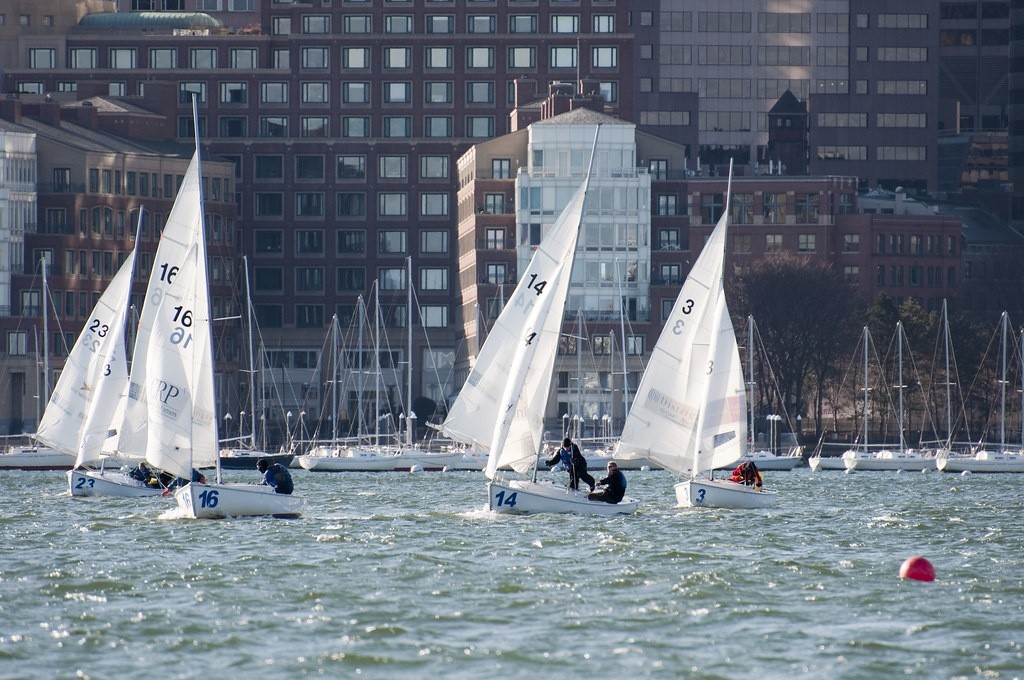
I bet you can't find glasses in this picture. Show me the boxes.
[608,466,614,469]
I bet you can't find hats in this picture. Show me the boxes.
[563,438,571,446]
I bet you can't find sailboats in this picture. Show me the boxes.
[215,255,973,473]
[612,158,779,508]
[1,258,148,469]
[99,93,309,519]
[935,311,1024,472]
[32,204,177,498]
[439,121,639,515]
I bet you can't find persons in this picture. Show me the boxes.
[545,438,595,492]
[257,459,294,495]
[161,468,206,497]
[731,461,763,487]
[130,462,172,489]
[588,461,627,504]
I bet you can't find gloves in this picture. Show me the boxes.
[571,458,576,463]
[544,461,550,466]
